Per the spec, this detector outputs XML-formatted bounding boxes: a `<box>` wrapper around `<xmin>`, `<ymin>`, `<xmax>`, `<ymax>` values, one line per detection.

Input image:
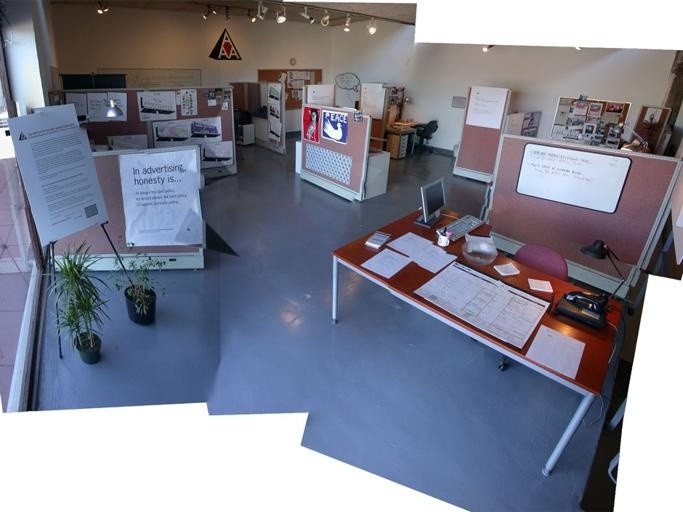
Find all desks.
<box><xmin>385</xmin><ymin>120</ymin><xmax>420</xmax><ymax>158</ymax></box>
<box><xmin>331</xmin><ymin>207</ymin><xmax>623</xmax><ymax>479</ymax></box>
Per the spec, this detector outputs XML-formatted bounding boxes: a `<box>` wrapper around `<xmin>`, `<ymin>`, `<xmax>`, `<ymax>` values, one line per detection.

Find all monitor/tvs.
<box><xmin>412</xmin><ymin>177</ymin><xmax>446</xmax><ymax>228</ymax></box>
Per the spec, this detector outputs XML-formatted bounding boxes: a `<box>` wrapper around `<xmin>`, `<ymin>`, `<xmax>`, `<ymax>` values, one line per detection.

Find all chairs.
<box><xmin>414</xmin><ymin>120</ymin><xmax>437</xmax><ymax>154</ymax></box>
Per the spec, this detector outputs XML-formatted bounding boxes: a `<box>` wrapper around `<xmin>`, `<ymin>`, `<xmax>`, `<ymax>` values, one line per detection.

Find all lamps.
<box><xmin>204</xmin><ymin>0</ymin><xmax>376</xmax><ymax>35</ymax></box>
<box><xmin>77</xmin><ymin>99</ymin><xmax>123</xmax><ymax>121</ymax></box>
<box><xmin>611</xmin><ymin>123</ymin><xmax>648</xmax><ymax>152</ymax></box>
<box><xmin>581</xmin><ymin>241</ymin><xmax>625</xmax><ymax>304</ymax></box>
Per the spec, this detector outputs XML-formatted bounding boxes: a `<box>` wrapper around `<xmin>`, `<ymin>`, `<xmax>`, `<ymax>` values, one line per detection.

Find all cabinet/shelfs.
<box><xmin>238</xmin><ymin>124</ymin><xmax>255</xmax><ymax>145</ymax></box>
<box><xmin>386</xmin><ymin>134</ymin><xmax>408</xmax><ymax>160</ymax></box>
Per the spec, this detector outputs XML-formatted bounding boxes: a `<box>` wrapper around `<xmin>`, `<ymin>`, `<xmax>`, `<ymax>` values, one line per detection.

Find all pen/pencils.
<box><xmin>436</xmin><ymin>226</ymin><xmax>453</xmax><ymax>237</ymax></box>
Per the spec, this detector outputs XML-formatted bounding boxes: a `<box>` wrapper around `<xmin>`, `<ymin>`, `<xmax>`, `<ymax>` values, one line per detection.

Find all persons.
<box><xmin>306</xmin><ymin>110</ymin><xmax>319</xmax><ymax>140</ymax></box>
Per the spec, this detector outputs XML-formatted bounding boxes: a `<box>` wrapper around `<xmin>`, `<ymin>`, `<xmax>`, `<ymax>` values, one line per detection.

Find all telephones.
<box><xmin>555</xmin><ymin>290</ymin><xmax>606</xmax><ymax>330</ymax></box>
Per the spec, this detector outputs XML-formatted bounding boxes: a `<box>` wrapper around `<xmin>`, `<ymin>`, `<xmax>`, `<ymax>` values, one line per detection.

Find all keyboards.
<box><xmin>436</xmin><ymin>214</ymin><xmax>485</xmax><ymax>242</ymax></box>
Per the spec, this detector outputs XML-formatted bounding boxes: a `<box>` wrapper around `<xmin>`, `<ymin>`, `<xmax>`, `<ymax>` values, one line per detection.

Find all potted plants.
<box><xmin>41</xmin><ymin>236</ymin><xmax>114</xmax><ymax>364</ymax></box>
<box><xmin>104</xmin><ymin>236</ymin><xmax>177</xmax><ymax>324</ymax></box>
